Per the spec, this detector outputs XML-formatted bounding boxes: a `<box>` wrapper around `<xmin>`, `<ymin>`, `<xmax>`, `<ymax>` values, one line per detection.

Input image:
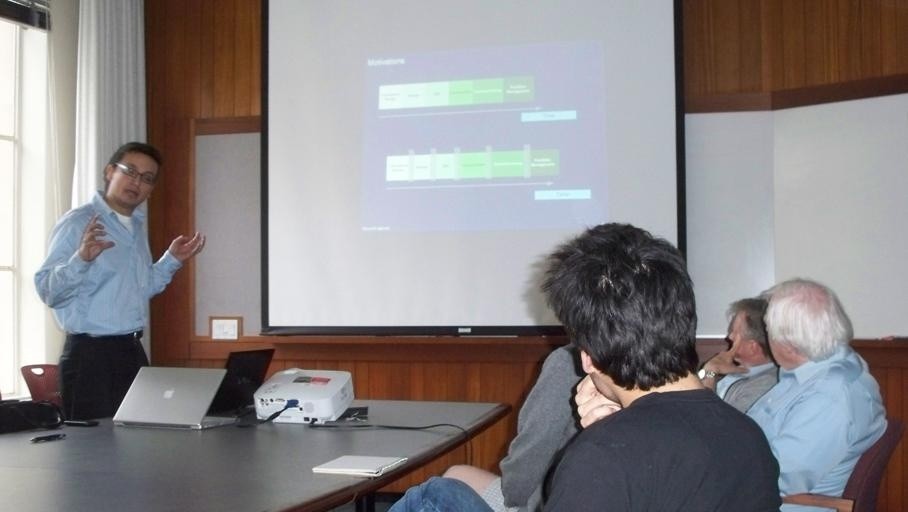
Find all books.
<box><xmin>313</xmin><ymin>455</ymin><xmax>411</xmax><ymax>480</ymax></box>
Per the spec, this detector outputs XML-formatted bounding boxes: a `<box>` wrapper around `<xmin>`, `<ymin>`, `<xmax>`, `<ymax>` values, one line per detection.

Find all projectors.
<box><xmin>253</xmin><ymin>368</ymin><xmax>354</xmax><ymax>425</ymax></box>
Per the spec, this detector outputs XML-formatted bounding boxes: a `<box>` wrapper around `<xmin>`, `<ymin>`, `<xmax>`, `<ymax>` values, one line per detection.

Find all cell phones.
<box><xmin>63</xmin><ymin>419</ymin><xmax>101</xmax><ymax>427</ymax></box>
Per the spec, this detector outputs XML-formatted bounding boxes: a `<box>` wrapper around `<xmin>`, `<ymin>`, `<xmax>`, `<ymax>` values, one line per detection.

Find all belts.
<box><xmin>131</xmin><ymin>330</ymin><xmax>143</xmax><ymax>341</ymax></box>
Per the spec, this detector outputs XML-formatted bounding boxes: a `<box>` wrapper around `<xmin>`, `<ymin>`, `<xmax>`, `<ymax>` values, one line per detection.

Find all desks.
<box><xmin>0</xmin><ymin>395</ymin><xmax>512</xmax><ymax>510</ymax></box>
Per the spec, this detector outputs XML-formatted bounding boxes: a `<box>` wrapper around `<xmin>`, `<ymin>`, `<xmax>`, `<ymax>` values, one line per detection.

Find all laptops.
<box><xmin>210</xmin><ymin>348</ymin><xmax>275</xmax><ymax>413</ymax></box>
<box><xmin>112</xmin><ymin>367</ymin><xmax>240</xmax><ymax>429</ymax></box>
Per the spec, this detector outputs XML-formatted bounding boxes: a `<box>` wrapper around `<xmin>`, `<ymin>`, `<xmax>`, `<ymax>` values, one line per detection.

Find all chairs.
<box><xmin>777</xmin><ymin>420</ymin><xmax>904</xmax><ymax>512</ymax></box>
<box><xmin>21</xmin><ymin>363</ymin><xmax>61</xmax><ymax>406</ymax></box>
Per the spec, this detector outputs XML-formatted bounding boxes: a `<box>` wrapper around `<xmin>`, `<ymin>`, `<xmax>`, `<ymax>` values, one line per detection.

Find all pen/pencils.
<box><xmin>30</xmin><ymin>433</ymin><xmax>66</xmax><ymax>442</ymax></box>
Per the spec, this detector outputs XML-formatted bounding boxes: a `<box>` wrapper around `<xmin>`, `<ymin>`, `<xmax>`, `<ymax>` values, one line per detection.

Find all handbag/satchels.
<box><xmin>0</xmin><ymin>400</ymin><xmax>65</xmax><ymax>434</ymax></box>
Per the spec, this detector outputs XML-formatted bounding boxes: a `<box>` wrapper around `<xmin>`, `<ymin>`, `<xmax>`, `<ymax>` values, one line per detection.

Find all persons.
<box><xmin>388</xmin><ymin>219</ymin><xmax>781</xmax><ymax>512</ymax></box>
<box><xmin>33</xmin><ymin>142</ymin><xmax>207</xmax><ymax>420</ymax></box>
<box><xmin>697</xmin><ymin>279</ymin><xmax>887</xmax><ymax>512</ymax></box>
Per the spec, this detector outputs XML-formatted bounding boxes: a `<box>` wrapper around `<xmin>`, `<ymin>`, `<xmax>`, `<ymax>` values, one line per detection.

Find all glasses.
<box><xmin>115</xmin><ymin>162</ymin><xmax>155</xmax><ymax>185</ymax></box>
